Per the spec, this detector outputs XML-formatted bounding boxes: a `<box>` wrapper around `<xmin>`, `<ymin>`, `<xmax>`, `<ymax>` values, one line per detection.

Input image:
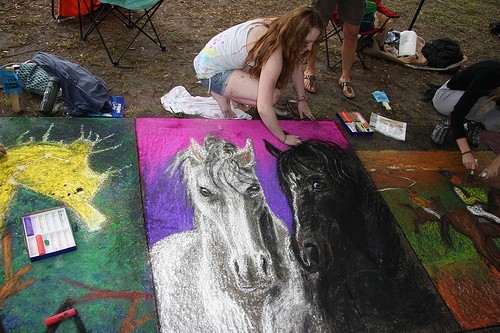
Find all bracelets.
<box><xmin>282</xmin><ymin>135</ymin><xmax>287</xmax><ymax>143</ymax></box>
<box><xmin>297</xmin><ymin>96</ymin><xmax>308</xmax><ymax>102</ymax></box>
<box><xmin>461</xmin><ymin>151</ymin><xmax>472</xmax><ymax>156</ymax></box>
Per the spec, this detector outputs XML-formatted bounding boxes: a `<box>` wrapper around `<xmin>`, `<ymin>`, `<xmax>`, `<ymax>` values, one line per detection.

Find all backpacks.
<box><xmin>420</xmin><ymin>37</ymin><xmax>464</xmax><ymax>68</ymax></box>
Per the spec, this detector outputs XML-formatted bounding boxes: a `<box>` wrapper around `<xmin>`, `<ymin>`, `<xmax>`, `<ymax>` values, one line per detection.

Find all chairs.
<box><xmin>52</xmin><ymin>0</ymin><xmax>166</xmax><ymax>66</ymax></box>
<box><xmin>317</xmin><ymin>0</ymin><xmax>401</xmax><ymax>70</ymax></box>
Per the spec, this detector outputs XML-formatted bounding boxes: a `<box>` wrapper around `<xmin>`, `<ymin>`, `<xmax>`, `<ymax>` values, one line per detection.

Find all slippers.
<box><xmin>303</xmin><ymin>69</ymin><xmax>317</xmax><ymax>93</ymax></box>
<box><xmin>338</xmin><ymin>80</ymin><xmax>355</xmax><ymax>98</ymax></box>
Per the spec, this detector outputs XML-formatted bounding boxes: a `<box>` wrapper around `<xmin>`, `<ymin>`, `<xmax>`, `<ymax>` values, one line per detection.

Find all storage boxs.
<box><xmin>87</xmin><ymin>96</ymin><xmax>124</xmax><ymax>118</ymax></box>
<box><xmin>337</xmin><ymin>112</ymin><xmax>374</xmax><ymax>136</ymax></box>
<box><xmin>20</xmin><ymin>206</ymin><xmax>77</xmax><ymax>262</ymax></box>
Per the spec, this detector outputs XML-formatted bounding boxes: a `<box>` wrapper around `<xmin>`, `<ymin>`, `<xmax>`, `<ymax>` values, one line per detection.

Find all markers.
<box><xmin>45</xmin><ymin>308</ymin><xmax>77</xmax><ymax>327</ymax></box>
<box><xmin>36</xmin><ymin>235</ymin><xmax>45</xmax><ymax>254</ymax></box>
<box><xmin>341</xmin><ymin>111</ymin><xmax>369</xmax><ymax>132</ymax></box>
<box><xmin>470</xmin><ymin>158</ymin><xmax>477</xmax><ymax>175</ymax></box>
<box><xmin>24</xmin><ymin>217</ymin><xmax>34</xmax><ymax>236</ymax></box>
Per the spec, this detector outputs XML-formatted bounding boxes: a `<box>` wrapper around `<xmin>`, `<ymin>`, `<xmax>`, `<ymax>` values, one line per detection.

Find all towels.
<box><xmin>162</xmin><ymin>85</ymin><xmax>251</xmax><ymax>120</ymax></box>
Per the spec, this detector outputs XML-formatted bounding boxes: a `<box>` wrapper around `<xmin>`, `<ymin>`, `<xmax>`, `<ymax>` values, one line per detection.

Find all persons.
<box><xmin>192</xmin><ymin>5</ymin><xmax>329</xmax><ymax>146</ymax></box>
<box><xmin>300</xmin><ymin>0</ymin><xmax>372</xmax><ymax>98</ymax></box>
<box><xmin>433</xmin><ymin>60</ymin><xmax>500</xmax><ymax>182</ymax></box>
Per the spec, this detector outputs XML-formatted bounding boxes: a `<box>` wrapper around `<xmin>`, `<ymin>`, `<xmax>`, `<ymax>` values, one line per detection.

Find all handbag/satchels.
<box><xmin>18</xmin><ymin>62</ymin><xmax>63</xmax><ymax>97</ymax></box>
